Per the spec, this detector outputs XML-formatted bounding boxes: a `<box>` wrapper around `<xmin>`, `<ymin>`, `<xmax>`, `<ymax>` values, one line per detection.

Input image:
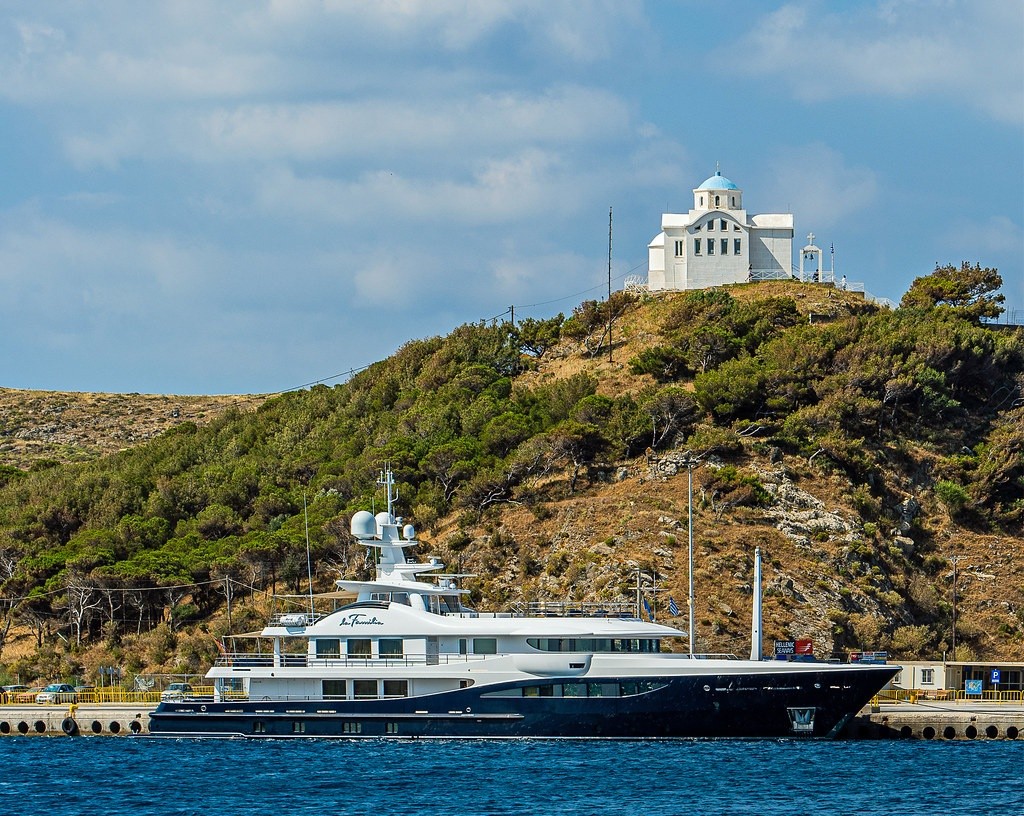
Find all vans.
<box><xmin>2</xmin><ymin>685</ymin><xmax>31</xmax><ymax>701</ymax></box>
<box><xmin>73</xmin><ymin>685</ymin><xmax>96</xmax><ymax>695</ymax></box>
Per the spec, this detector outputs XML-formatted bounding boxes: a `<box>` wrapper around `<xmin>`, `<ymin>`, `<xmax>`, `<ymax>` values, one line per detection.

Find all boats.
<box><xmin>142</xmin><ymin>457</ymin><xmax>903</xmax><ymax>740</ymax></box>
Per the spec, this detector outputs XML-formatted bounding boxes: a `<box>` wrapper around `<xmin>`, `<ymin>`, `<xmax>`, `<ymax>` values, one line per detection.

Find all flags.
<box><xmin>669</xmin><ymin>596</ymin><xmax>678</xmax><ymax>617</ymax></box>
<box><xmin>643</xmin><ymin>598</ymin><xmax>654</xmax><ymax>621</ymax></box>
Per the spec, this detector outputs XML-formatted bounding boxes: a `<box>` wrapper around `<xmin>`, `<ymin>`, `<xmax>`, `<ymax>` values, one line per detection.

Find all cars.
<box><xmin>16</xmin><ymin>686</ymin><xmax>44</xmax><ymax>702</ymax></box>
<box><xmin>160</xmin><ymin>683</ymin><xmax>194</xmax><ymax>702</ymax></box>
<box><xmin>36</xmin><ymin>684</ymin><xmax>79</xmax><ymax>705</ymax></box>
